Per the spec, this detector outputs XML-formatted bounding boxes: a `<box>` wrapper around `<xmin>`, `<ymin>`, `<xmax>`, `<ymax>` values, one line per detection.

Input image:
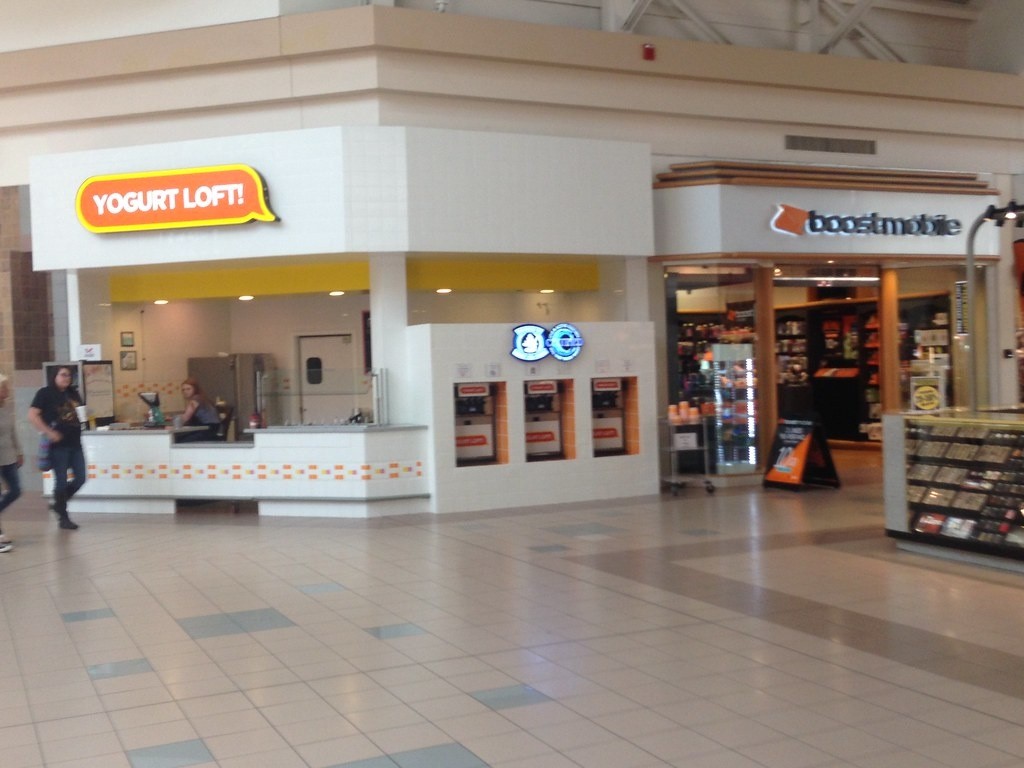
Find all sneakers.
<box><xmin>0</xmin><ymin>542</ymin><xmax>13</xmax><ymax>552</ymax></box>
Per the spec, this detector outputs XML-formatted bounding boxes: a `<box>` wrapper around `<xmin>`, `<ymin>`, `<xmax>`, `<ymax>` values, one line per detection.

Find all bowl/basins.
<box><xmin>668</xmin><ymin>401</ymin><xmax>701</xmax><ymax>424</ymax></box>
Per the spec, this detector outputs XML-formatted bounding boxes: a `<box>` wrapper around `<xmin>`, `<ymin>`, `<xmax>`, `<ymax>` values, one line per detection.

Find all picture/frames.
<box><xmin>120</xmin><ymin>331</ymin><xmax>134</xmax><ymax>347</ymax></box>
<box><xmin>120</xmin><ymin>351</ymin><xmax>137</xmax><ymax>370</ymax></box>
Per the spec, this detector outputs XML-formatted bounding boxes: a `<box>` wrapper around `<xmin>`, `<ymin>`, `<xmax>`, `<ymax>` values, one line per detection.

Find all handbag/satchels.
<box><xmin>38</xmin><ymin>432</ymin><xmax>52</xmax><ymax>471</ymax></box>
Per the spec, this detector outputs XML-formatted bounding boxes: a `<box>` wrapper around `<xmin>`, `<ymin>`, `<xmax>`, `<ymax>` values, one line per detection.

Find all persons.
<box><xmin>164</xmin><ymin>378</ymin><xmax>219</xmax><ymax>443</ymax></box>
<box><xmin>28</xmin><ymin>364</ymin><xmax>85</xmax><ymax>529</ymax></box>
<box><xmin>0</xmin><ymin>375</ymin><xmax>24</xmax><ymax>552</ymax></box>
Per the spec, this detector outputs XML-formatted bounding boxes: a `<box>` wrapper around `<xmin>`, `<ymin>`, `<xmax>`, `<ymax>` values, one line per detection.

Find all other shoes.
<box><xmin>54</xmin><ymin>503</ymin><xmax>69</xmax><ymax>519</ymax></box>
<box><xmin>59</xmin><ymin>521</ymin><xmax>77</xmax><ymax>529</ymax></box>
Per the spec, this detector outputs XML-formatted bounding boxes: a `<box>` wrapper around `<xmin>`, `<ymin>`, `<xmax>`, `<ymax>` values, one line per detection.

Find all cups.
<box><xmin>247</xmin><ymin>415</ymin><xmax>260</xmax><ymax>428</ymax></box>
<box><xmin>75</xmin><ymin>405</ymin><xmax>88</xmax><ymax>423</ymax></box>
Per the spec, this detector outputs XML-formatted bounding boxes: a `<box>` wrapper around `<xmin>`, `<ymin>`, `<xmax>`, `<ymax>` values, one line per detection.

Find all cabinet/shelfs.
<box><xmin>659</xmin><ymin>417</ymin><xmax>715</xmax><ymax>495</ymax></box>
